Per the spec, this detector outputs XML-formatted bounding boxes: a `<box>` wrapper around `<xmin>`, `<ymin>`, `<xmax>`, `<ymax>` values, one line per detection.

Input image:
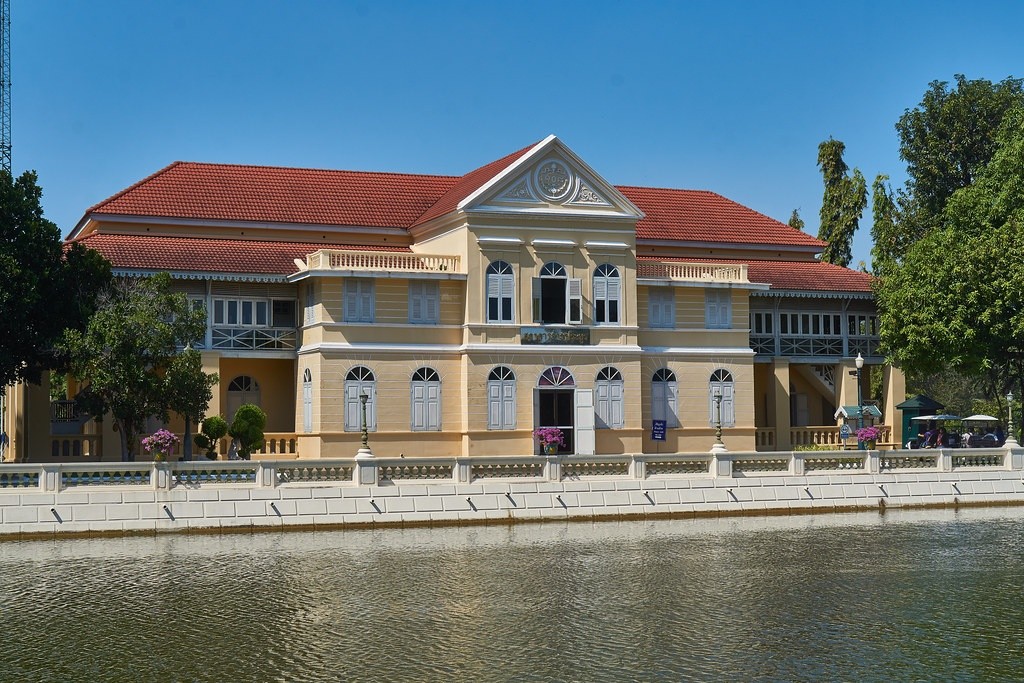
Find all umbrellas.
<box><xmin>912</xmin><ymin>414</ymin><xmax>961</xmax><ymax>420</ymax></box>
<box><xmin>961</xmin><ymin>414</ymin><xmax>998</xmax><ymax>421</ymax></box>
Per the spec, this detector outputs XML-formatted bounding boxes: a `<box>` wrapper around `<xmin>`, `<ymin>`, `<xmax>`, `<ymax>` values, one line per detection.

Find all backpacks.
<box><xmin>965</xmin><ymin>432</ymin><xmax>982</xmax><ymax>448</ymax></box>
<box><xmin>982</xmin><ymin>434</ymin><xmax>996</xmax><ymax>448</ymax></box>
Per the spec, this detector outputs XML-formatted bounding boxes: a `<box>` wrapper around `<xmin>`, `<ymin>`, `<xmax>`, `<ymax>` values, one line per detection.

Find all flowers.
<box><xmin>532</xmin><ymin>428</ymin><xmax>566</xmax><ymax>448</ymax></box>
<box><xmin>141</xmin><ymin>428</ymin><xmax>180</xmax><ymax>457</ymax></box>
<box><xmin>855</xmin><ymin>427</ymin><xmax>882</xmax><ymax>442</ymax></box>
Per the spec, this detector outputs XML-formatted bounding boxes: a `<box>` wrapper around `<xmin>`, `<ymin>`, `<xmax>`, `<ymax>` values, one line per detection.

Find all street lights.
<box><xmin>855</xmin><ymin>351</ymin><xmax>865</xmax><ymax>450</ymax></box>
<box><xmin>183</xmin><ymin>342</ymin><xmax>194</xmax><ymax>459</ymax></box>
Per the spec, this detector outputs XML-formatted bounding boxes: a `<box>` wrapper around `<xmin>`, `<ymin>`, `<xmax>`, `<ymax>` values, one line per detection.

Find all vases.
<box><xmin>154</xmin><ymin>452</ymin><xmax>167</xmax><ymax>461</ymax></box>
<box><xmin>861</xmin><ymin>440</ymin><xmax>876</xmax><ymax>450</ymax></box>
<box><xmin>542</xmin><ymin>442</ymin><xmax>558</xmax><ymax>455</ymax></box>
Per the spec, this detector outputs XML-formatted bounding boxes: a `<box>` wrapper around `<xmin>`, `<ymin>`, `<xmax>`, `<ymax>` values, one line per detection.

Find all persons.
<box><xmin>923</xmin><ymin>422</ymin><xmax>958</xmax><ymax>447</ymax></box>
<box><xmin>964</xmin><ymin>426</ymin><xmax>1004</xmax><ymax>447</ymax></box>
